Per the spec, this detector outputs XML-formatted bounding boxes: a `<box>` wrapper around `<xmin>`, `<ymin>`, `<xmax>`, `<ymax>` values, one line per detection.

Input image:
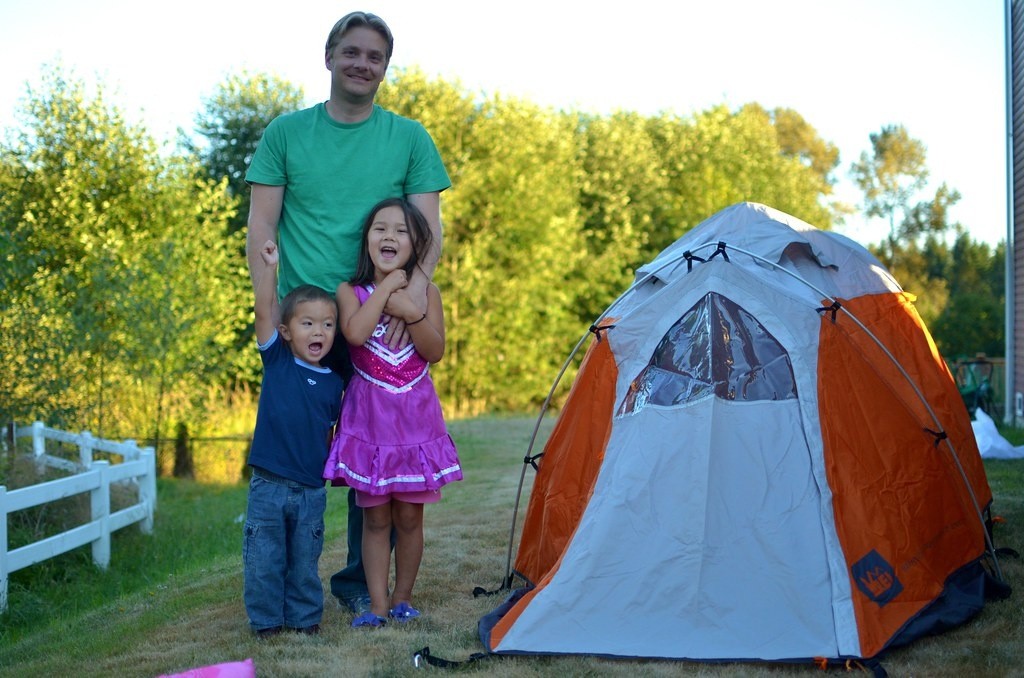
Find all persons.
<box><xmin>241</xmin><ymin>239</ymin><xmax>346</xmax><ymax>641</ymax></box>
<box><xmin>241</xmin><ymin>12</ymin><xmax>451</xmax><ymax>614</ymax></box>
<box><xmin>317</xmin><ymin>195</ymin><xmax>462</xmax><ymax>635</ymax></box>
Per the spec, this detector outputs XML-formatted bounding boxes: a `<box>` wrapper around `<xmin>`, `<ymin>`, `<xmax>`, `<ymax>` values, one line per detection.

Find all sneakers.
<box><xmin>293</xmin><ymin>623</ymin><xmax>323</xmax><ymax>636</ymax></box>
<box><xmin>256</xmin><ymin>626</ymin><xmax>281</xmax><ymax>640</ymax></box>
<box><xmin>345</xmin><ymin>595</ymin><xmax>371</xmax><ymax>617</ymax></box>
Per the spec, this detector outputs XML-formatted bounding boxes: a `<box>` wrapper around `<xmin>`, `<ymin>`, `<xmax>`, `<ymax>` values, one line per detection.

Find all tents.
<box><xmin>474</xmin><ymin>198</ymin><xmax>1012</xmax><ymax>668</ymax></box>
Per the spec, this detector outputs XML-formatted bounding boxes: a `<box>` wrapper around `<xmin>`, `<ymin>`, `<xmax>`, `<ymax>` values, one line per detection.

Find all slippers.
<box><xmin>351</xmin><ymin>612</ymin><xmax>387</xmax><ymax>628</ymax></box>
<box><xmin>388</xmin><ymin>603</ymin><xmax>420</xmax><ymax>624</ymax></box>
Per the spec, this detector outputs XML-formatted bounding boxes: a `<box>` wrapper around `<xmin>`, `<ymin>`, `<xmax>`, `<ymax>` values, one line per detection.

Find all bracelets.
<box><xmin>406</xmin><ymin>313</ymin><xmax>426</xmax><ymax>326</ymax></box>
